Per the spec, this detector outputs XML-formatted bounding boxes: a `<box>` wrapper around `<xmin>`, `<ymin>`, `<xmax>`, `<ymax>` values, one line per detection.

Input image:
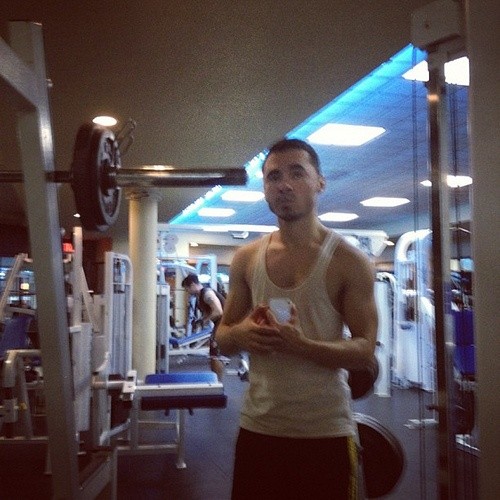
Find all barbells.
<box><xmin>1</xmin><ymin>118</ymin><xmax>247</xmax><ymax>232</ymax></box>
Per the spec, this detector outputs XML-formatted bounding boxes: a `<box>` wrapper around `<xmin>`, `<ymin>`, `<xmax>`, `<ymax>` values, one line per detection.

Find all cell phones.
<box><xmin>268</xmin><ymin>296</ymin><xmax>292</xmax><ymax>323</ymax></box>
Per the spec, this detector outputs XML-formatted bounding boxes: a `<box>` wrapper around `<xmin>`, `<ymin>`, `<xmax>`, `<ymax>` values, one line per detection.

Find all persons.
<box><xmin>0</xmin><ymin>301</ymin><xmax>32</xmax><ymax>406</ymax></box>
<box><xmin>182</xmin><ymin>272</ymin><xmax>226</xmax><ymax>383</ymax></box>
<box><xmin>215</xmin><ymin>137</ymin><xmax>379</xmax><ymax>500</ymax></box>
